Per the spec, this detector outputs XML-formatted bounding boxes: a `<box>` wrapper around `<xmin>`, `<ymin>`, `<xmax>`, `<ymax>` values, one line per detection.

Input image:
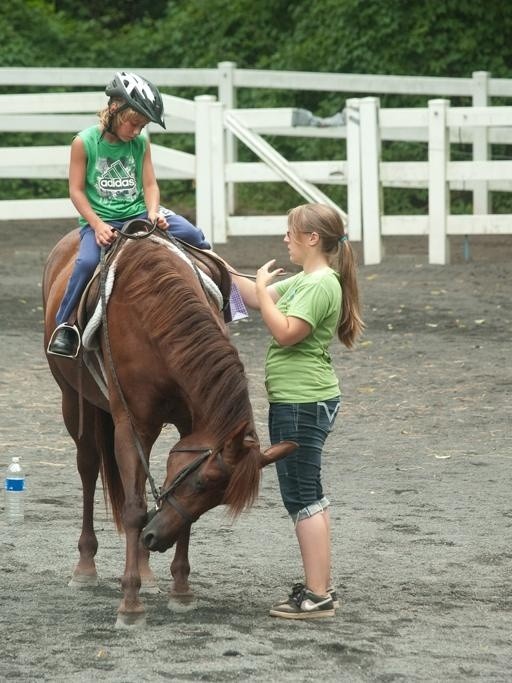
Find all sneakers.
<box><xmin>270</xmin><ymin>589</ymin><xmax>338</xmax><ymax>619</ymax></box>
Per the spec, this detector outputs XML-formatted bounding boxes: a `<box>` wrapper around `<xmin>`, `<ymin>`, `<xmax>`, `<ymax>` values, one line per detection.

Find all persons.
<box><xmin>49</xmin><ymin>70</ymin><xmax>214</xmax><ymax>357</ymax></box>
<box><xmin>194</xmin><ymin>203</ymin><xmax>368</xmax><ymax>618</ymax></box>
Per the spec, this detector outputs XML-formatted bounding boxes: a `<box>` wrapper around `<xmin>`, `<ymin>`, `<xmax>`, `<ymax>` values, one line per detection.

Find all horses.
<box><xmin>41</xmin><ymin>224</ymin><xmax>300</xmax><ymax>632</ymax></box>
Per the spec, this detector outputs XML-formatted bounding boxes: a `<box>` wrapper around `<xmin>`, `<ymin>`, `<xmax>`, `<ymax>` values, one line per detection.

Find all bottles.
<box><xmin>463</xmin><ymin>233</ymin><xmax>471</xmax><ymax>263</ymax></box>
<box><xmin>5</xmin><ymin>456</ymin><xmax>25</xmax><ymax>523</ymax></box>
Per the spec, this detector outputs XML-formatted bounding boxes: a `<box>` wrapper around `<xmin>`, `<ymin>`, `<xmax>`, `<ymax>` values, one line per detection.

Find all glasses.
<box><xmin>287</xmin><ymin>231</ymin><xmax>313</xmax><ymax>238</ymax></box>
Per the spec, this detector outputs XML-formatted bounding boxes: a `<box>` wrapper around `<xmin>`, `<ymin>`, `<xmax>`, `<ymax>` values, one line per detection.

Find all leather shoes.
<box><xmin>51</xmin><ymin>329</ymin><xmax>75</xmax><ymax>355</ymax></box>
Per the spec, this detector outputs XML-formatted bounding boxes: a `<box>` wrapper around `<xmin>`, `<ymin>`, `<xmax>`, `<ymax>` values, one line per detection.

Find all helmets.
<box><xmin>105</xmin><ymin>72</ymin><xmax>166</xmax><ymax>130</ymax></box>
